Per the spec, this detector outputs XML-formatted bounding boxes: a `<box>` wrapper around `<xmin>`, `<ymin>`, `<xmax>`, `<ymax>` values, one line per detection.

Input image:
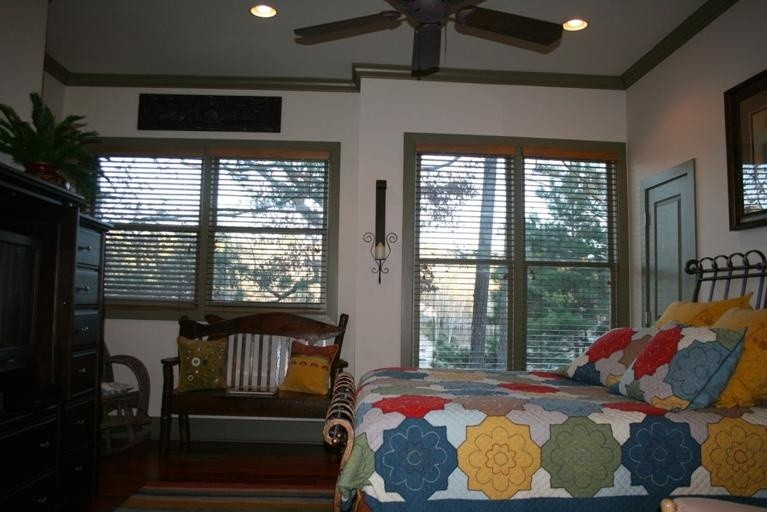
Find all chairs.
<box><xmin>98</xmin><ymin>344</ymin><xmax>152</xmax><ymax>455</ymax></box>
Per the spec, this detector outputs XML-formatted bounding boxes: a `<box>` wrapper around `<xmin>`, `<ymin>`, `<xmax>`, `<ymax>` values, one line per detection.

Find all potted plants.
<box><xmin>0</xmin><ymin>91</ymin><xmax>111</xmax><ymax>188</ymax></box>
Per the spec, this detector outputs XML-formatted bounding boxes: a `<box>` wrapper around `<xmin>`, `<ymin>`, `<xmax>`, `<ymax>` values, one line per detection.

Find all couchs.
<box><xmin>161</xmin><ymin>313</ymin><xmax>349</xmax><ymax>454</ymax></box>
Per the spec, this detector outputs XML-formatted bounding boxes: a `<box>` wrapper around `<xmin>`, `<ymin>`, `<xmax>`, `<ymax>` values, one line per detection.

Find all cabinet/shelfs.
<box><xmin>1</xmin><ymin>403</ymin><xmax>60</xmax><ymax>510</ymax></box>
<box><xmin>60</xmin><ymin>213</ymin><xmax>116</xmax><ymax>511</ymax></box>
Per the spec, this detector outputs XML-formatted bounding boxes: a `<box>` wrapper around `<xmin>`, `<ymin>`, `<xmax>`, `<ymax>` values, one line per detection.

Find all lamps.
<box><xmin>562</xmin><ymin>17</ymin><xmax>590</xmax><ymax>33</ymax></box>
<box><xmin>247</xmin><ymin>3</ymin><xmax>279</xmax><ymax>19</ymax></box>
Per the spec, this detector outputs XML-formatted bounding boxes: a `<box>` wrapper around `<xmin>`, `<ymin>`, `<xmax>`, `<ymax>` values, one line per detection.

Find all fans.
<box><xmin>294</xmin><ymin>0</ymin><xmax>562</xmax><ymax>77</ymax></box>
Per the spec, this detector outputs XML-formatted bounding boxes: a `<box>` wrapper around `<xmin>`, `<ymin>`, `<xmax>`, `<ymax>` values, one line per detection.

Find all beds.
<box><xmin>323</xmin><ymin>251</ymin><xmax>767</xmax><ymax>512</ymax></box>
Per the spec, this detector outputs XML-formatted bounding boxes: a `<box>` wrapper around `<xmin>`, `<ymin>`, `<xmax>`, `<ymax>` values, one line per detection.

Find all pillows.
<box><xmin>557</xmin><ymin>327</ymin><xmax>659</xmax><ymax>388</ymax></box>
<box><xmin>655</xmin><ymin>292</ymin><xmax>754</xmax><ymax>327</ymax></box>
<box><xmin>176</xmin><ymin>336</ymin><xmax>229</xmax><ymax>392</ymax></box>
<box><xmin>607</xmin><ymin>321</ymin><xmax>748</xmax><ymax>413</ymax></box>
<box><xmin>711</xmin><ymin>307</ymin><xmax>767</xmax><ymax>408</ymax></box>
<box><xmin>280</xmin><ymin>340</ymin><xmax>338</xmax><ymax>396</ymax></box>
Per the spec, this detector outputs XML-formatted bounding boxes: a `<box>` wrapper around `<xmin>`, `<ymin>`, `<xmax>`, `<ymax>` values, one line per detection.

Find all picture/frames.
<box><xmin>724</xmin><ymin>69</ymin><xmax>767</xmax><ymax>231</ymax></box>
